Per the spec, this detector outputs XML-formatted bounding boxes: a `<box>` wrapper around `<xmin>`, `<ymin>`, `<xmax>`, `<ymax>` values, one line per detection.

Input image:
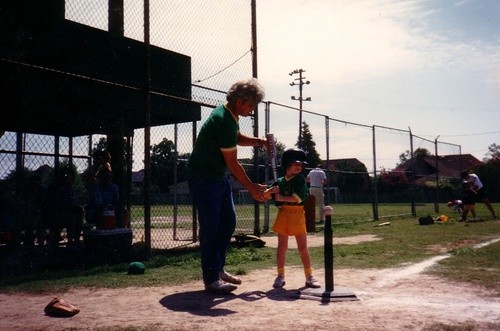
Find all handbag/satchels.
<box><xmin>419</xmin><ymin>215</ymin><xmax>435</xmax><ymax>225</ymax></box>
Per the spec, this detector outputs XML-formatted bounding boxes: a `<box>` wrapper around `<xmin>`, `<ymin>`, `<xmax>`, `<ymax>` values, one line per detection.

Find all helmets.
<box><xmin>281</xmin><ymin>149</ymin><xmax>308</xmax><ymax>176</ymax></box>
<box><xmin>127</xmin><ymin>261</ymin><xmax>146</xmax><ymax>276</ymax></box>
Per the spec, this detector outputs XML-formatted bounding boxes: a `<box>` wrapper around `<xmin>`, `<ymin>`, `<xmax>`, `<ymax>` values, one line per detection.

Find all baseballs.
<box><xmin>324</xmin><ymin>206</ymin><xmax>333</xmax><ymax>215</ymax></box>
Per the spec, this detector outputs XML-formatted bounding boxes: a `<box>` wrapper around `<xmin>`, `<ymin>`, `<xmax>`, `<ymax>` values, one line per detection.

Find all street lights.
<box><xmin>289</xmin><ymin>69</ymin><xmax>311</xmax><ymax>148</ymax></box>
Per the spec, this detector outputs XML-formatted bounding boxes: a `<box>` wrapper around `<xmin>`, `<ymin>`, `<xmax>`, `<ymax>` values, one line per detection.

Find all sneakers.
<box><xmin>273</xmin><ymin>277</ymin><xmax>285</xmax><ymax>287</ymax></box>
<box><xmin>306</xmin><ymin>274</ymin><xmax>320</xmax><ymax>288</ymax></box>
<box><xmin>219</xmin><ymin>271</ymin><xmax>242</xmax><ymax>284</ymax></box>
<box><xmin>205</xmin><ymin>279</ymin><xmax>237</xmax><ymax>294</ymax></box>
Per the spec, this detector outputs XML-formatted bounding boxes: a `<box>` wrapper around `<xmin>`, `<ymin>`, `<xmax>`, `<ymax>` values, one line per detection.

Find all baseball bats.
<box><xmin>266</xmin><ymin>133</ymin><xmax>282</xmax><ymax>210</ymax></box>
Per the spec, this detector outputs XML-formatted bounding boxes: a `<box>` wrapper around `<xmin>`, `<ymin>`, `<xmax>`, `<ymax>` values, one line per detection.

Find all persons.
<box><xmin>305</xmin><ymin>163</ymin><xmax>328</xmax><ymax>222</ymax></box>
<box><xmin>262</xmin><ymin>147</ymin><xmax>321</xmax><ymax>288</ymax></box>
<box><xmin>32</xmin><ymin>164</ymin><xmax>53</xmax><ymax>182</ymax></box>
<box><xmin>447</xmin><ymin>199</ymin><xmax>463</xmax><ymax>215</ymax></box>
<box><xmin>82</xmin><ymin>149</ymin><xmax>114</xmax><ymax>186</ymax></box>
<box><xmin>460</xmin><ymin>181</ymin><xmax>478</xmax><ymax>222</ymax></box>
<box><xmin>185</xmin><ymin>76</ymin><xmax>277</xmax><ymax>295</ymax></box>
<box><xmin>85</xmin><ymin>163</ymin><xmax>124</xmax><ymax>225</ymax></box>
<box><xmin>458</xmin><ymin>170</ymin><xmax>497</xmax><ymax>223</ymax></box>
